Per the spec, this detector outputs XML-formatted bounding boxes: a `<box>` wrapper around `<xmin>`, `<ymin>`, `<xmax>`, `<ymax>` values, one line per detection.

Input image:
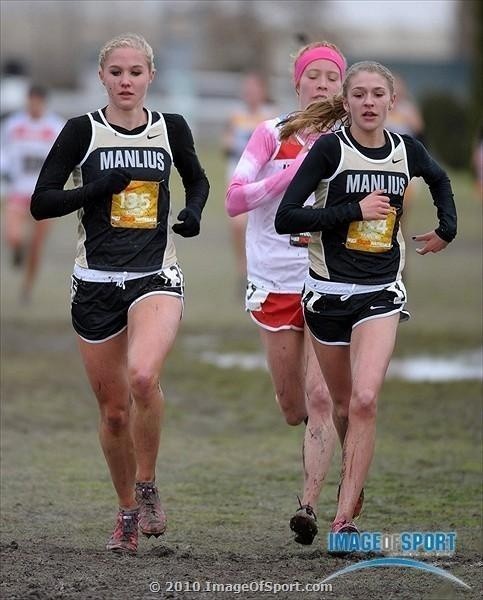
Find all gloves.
<box><xmin>172</xmin><ymin>209</ymin><xmax>201</xmax><ymax>237</ymax></box>
<box><xmin>93</xmin><ymin>168</ymin><xmax>131</xmax><ymax>194</ymax></box>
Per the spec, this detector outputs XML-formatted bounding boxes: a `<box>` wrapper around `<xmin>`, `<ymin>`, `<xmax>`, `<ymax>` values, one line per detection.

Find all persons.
<box><xmin>216</xmin><ymin>72</ymin><xmax>283</xmax><ymax>291</ymax></box>
<box><xmin>221</xmin><ymin>40</ymin><xmax>356</xmax><ymax>551</ymax></box>
<box><xmin>1</xmin><ymin>82</ymin><xmax>70</xmax><ymax>307</ymax></box>
<box><xmin>269</xmin><ymin>57</ymin><xmax>460</xmax><ymax>560</ymax></box>
<box><xmin>383</xmin><ymin>76</ymin><xmax>425</xmax><ymax>230</ymax></box>
<box><xmin>28</xmin><ymin>31</ymin><xmax>211</xmax><ymax>558</ymax></box>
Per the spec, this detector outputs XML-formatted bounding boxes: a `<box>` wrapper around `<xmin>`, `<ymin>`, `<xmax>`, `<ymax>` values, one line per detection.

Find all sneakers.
<box><xmin>106</xmin><ymin>510</ymin><xmax>138</xmax><ymax>556</ymax></box>
<box><xmin>290</xmin><ymin>509</ymin><xmax>317</xmax><ymax>545</ymax></box>
<box><xmin>135</xmin><ymin>481</ymin><xmax>166</xmax><ymax>538</ymax></box>
<box><xmin>330</xmin><ymin>522</ymin><xmax>359</xmax><ymax>555</ymax></box>
<box><xmin>338</xmin><ymin>485</ymin><xmax>364</xmax><ymax>519</ymax></box>
